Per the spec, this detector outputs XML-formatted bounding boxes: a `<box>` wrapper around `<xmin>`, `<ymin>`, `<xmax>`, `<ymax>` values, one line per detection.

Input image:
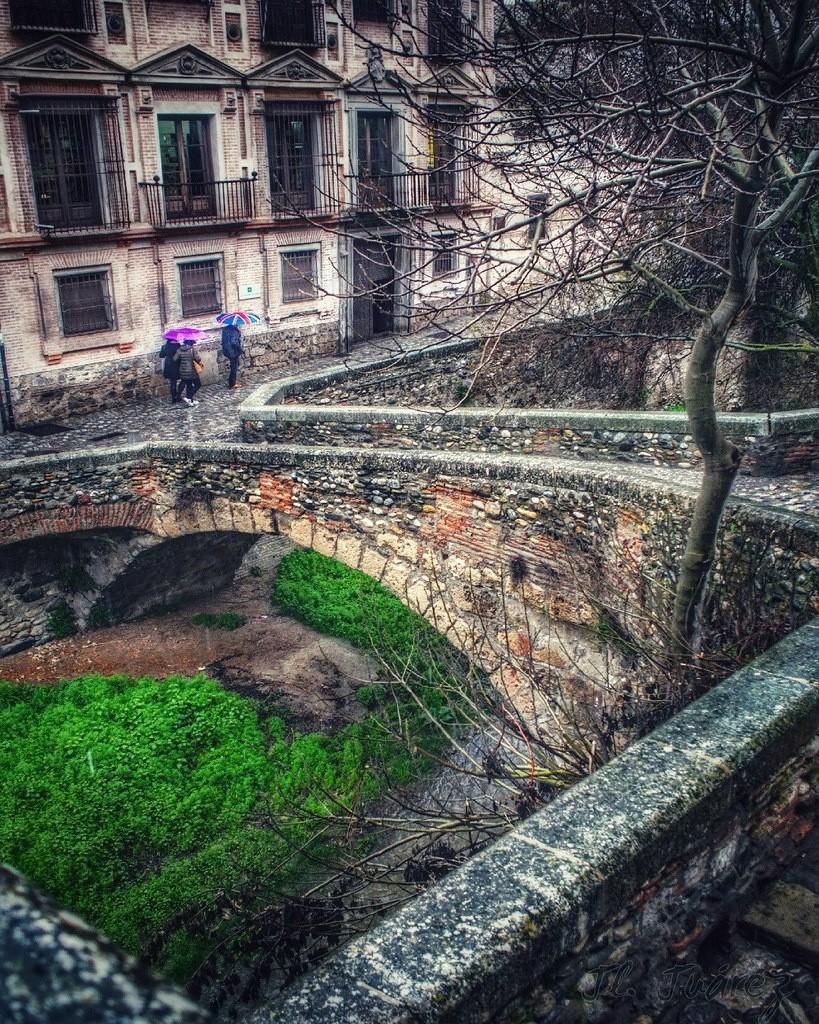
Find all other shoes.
<box><xmin>193</xmin><ymin>400</ymin><xmax>199</xmax><ymax>405</ymax></box>
<box><xmin>172</xmin><ymin>400</ymin><xmax>176</xmax><ymax>405</ymax></box>
<box><xmin>231</xmin><ymin>384</ymin><xmax>241</xmax><ymax>388</ymax></box>
<box><xmin>183</xmin><ymin>398</ymin><xmax>194</xmax><ymax>406</ymax></box>
<box><xmin>177</xmin><ymin>397</ymin><xmax>182</xmax><ymax>402</ymax></box>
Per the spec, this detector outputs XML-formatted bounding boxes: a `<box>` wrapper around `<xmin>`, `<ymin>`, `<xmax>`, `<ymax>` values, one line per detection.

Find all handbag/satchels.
<box><xmin>191</xmin><ymin>348</ymin><xmax>203</xmax><ymax>373</ymax></box>
<box><xmin>162</xmin><ymin>345</ymin><xmax>166</xmax><ymax>373</ymax></box>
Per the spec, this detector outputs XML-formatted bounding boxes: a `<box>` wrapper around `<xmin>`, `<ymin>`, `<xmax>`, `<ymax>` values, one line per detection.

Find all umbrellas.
<box><xmin>163</xmin><ymin>327</ymin><xmax>206</xmax><ymax>342</ymax></box>
<box><xmin>216</xmin><ymin>312</ymin><xmax>260</xmax><ymax>325</ymax></box>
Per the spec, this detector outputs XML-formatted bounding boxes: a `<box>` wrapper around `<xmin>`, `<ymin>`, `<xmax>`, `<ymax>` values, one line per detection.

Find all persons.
<box><xmin>173</xmin><ymin>340</ymin><xmax>201</xmax><ymax>406</ymax></box>
<box><xmin>159</xmin><ymin>339</ymin><xmax>186</xmax><ymax>402</ymax></box>
<box><xmin>221</xmin><ymin>325</ymin><xmax>243</xmax><ymax>387</ymax></box>
